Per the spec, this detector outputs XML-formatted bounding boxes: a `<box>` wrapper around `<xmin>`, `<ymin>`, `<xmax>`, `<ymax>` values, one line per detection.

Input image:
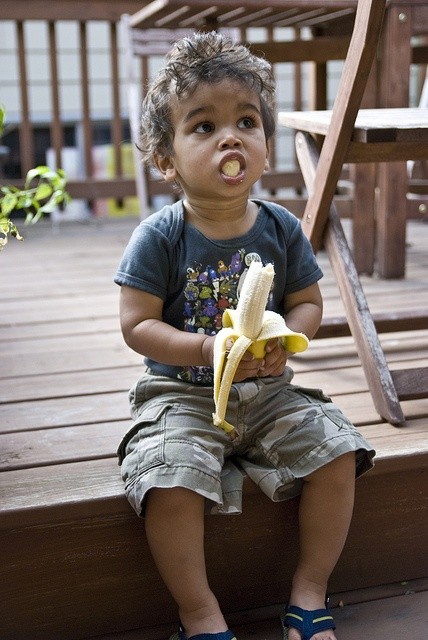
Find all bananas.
<box><xmin>210</xmin><ymin>258</ymin><xmax>310</xmax><ymax>442</ymax></box>
<box><xmin>220</xmin><ymin>158</ymin><xmax>242</xmax><ymax>178</ymax></box>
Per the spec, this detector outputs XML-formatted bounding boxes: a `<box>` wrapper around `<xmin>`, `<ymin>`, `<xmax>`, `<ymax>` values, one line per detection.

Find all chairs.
<box><xmin>277</xmin><ymin>0</ymin><xmax>428</xmax><ymax>426</ymax></box>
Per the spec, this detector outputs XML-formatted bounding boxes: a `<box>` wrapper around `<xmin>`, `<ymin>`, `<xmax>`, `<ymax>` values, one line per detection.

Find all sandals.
<box><xmin>281</xmin><ymin>604</ymin><xmax>335</xmax><ymax>640</ymax></box>
<box><xmin>178</xmin><ymin>626</ymin><xmax>237</xmax><ymax>639</ymax></box>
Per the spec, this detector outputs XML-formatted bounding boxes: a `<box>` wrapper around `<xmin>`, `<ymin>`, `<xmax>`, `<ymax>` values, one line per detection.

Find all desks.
<box><xmin>118</xmin><ymin>0</ymin><xmax>428</xmax><ymax>278</ymax></box>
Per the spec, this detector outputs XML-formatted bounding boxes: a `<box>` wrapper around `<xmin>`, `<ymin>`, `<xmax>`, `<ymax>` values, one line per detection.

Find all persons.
<box><xmin>113</xmin><ymin>31</ymin><xmax>378</xmax><ymax>639</ymax></box>
